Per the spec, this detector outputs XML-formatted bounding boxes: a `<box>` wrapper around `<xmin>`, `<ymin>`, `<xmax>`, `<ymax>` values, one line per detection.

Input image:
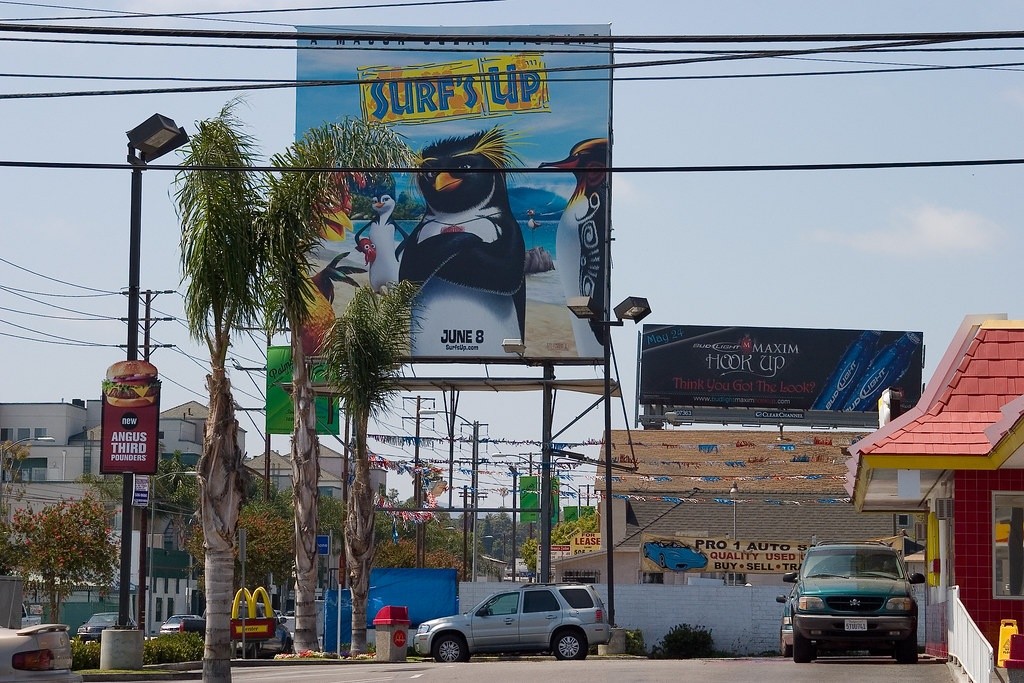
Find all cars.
<box><xmin>202</xmin><ymin>599</ymin><xmax>293</xmax><ymax>658</ymax></box>
<box><xmin>0</xmin><ymin>623</ymin><xmax>84</xmax><ymax>682</ymax></box>
<box><xmin>76</xmin><ymin>612</ymin><xmax>139</xmax><ymax>641</ymax></box>
<box><xmin>160</xmin><ymin>614</ymin><xmax>207</xmax><ymax>637</ymax></box>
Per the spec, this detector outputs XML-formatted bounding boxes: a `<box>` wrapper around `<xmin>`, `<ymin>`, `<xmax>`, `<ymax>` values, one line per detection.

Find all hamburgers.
<box><xmin>101</xmin><ymin>360</ymin><xmax>162</xmax><ymax>407</ymax></box>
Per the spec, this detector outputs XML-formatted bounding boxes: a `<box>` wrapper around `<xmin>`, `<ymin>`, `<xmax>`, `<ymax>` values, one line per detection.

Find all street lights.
<box><xmin>566</xmin><ymin>295</ymin><xmax>653</xmax><ymax>657</ymax></box>
<box><xmin>0</xmin><ymin>437</ymin><xmax>55</xmax><ymax>513</ymax></box>
<box><xmin>147</xmin><ymin>471</ymin><xmax>199</xmax><ymax>639</ymax></box>
<box><xmin>491</xmin><ymin>452</ymin><xmax>541</xmax><ymax>584</ymax></box>
<box><xmin>417</xmin><ymin>409</ymin><xmax>478</xmax><ymax>581</ymax></box>
<box><xmin>559</xmin><ymin>482</ymin><xmax>581</xmax><ymax>519</ymax></box>
<box><xmin>97</xmin><ymin>112</ymin><xmax>191</xmax><ymax>669</ymax></box>
<box><xmin>729</xmin><ymin>480</ymin><xmax>739</xmax><ymax>588</ymax></box>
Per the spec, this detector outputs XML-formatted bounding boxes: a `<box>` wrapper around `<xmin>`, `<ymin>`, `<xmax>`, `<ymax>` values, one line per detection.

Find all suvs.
<box><xmin>413</xmin><ymin>581</ymin><xmax>612</xmax><ymax>663</ymax></box>
<box><xmin>776</xmin><ymin>538</ymin><xmax>926</xmax><ymax>664</ymax></box>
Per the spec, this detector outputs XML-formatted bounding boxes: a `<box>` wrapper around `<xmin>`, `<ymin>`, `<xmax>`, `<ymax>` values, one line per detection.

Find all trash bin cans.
<box><xmin>372</xmin><ymin>605</ymin><xmax>411</xmax><ymax>662</ymax></box>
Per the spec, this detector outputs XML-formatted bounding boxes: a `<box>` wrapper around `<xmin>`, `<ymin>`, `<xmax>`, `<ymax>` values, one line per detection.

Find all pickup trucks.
<box><xmin>21</xmin><ymin>603</ymin><xmax>42</xmax><ymax>628</ymax></box>
